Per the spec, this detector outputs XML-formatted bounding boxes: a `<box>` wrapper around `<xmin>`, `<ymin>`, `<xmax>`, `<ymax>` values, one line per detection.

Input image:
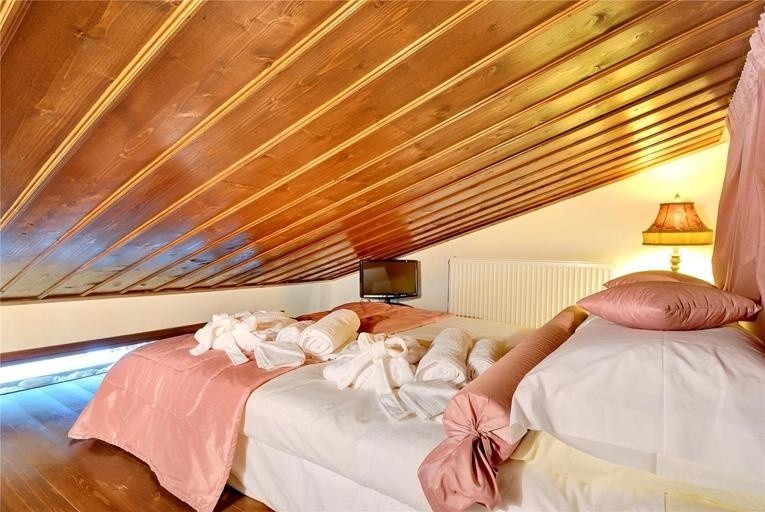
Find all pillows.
<box><xmin>575</xmin><ymin>281</ymin><xmax>762</xmax><ymax>331</ymax></box>
<box><xmin>602</xmin><ymin>269</ymin><xmax>720</xmax><ymax>289</ymax></box>
<box><xmin>508</xmin><ymin>312</ymin><xmax>765</xmax><ymax>508</ymax></box>
<box><xmin>417</xmin><ymin>305</ymin><xmax>589</xmax><ymax>509</ymax></box>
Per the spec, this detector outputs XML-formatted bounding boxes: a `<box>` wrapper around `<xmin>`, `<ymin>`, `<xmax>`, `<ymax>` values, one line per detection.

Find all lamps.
<box><xmin>640</xmin><ymin>203</ymin><xmax>715</xmax><ymax>276</ymax></box>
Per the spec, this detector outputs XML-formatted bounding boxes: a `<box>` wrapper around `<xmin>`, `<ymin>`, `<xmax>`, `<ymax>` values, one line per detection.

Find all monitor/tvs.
<box><xmin>359</xmin><ymin>259</ymin><xmax>418</xmax><ymax>308</ymax></box>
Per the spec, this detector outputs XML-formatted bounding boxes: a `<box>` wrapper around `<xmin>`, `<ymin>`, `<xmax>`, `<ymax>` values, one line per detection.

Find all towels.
<box><xmin>277</xmin><ymin>309</ymin><xmax>498</xmax><ymax>407</ymax></box>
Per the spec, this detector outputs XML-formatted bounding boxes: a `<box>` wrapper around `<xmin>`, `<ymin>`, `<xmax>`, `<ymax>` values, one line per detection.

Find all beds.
<box><xmin>69</xmin><ymin>301</ymin><xmax>765</xmax><ymax>509</ymax></box>
